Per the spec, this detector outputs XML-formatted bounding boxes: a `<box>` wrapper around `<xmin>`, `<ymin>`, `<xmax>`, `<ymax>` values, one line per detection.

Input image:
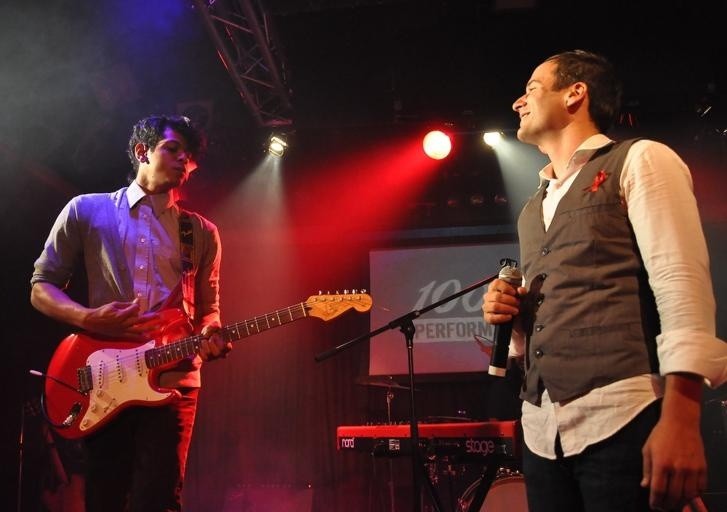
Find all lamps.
<box><xmin>259</xmin><ymin>130</ymin><xmax>291</xmax><ymax>162</ymax></box>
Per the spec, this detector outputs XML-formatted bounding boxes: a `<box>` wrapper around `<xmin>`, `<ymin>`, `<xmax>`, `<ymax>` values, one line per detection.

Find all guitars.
<box><xmin>45</xmin><ymin>288</ymin><xmax>373</xmax><ymax>440</ymax></box>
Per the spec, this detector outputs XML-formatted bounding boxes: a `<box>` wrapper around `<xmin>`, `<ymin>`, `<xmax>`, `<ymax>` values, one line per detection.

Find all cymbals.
<box><xmin>355</xmin><ymin>378</ymin><xmax>419</xmax><ymax>393</ymax></box>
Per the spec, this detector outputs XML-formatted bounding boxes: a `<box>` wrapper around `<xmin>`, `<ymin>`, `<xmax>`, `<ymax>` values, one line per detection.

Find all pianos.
<box><xmin>336</xmin><ymin>415</ymin><xmax>523</xmax><ymax>466</ymax></box>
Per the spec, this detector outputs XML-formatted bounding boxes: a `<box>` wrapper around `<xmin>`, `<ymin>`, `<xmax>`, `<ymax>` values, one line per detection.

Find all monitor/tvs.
<box><xmin>358</xmin><ymin>233</ymin><xmax>528</xmax><ymax>386</ymax></box>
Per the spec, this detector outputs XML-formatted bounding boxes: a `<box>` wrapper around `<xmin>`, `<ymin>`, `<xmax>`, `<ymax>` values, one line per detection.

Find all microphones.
<box><xmin>29</xmin><ymin>369</ymin><xmax>80</xmax><ymax>393</ymax></box>
<box><xmin>488</xmin><ymin>264</ymin><xmax>523</xmax><ymax>381</ymax></box>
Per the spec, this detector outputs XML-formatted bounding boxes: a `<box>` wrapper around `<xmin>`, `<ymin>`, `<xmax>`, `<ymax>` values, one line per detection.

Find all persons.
<box><xmin>26</xmin><ymin>115</ymin><xmax>234</xmax><ymax>510</ymax></box>
<box><xmin>479</xmin><ymin>48</ymin><xmax>726</xmax><ymax>510</ymax></box>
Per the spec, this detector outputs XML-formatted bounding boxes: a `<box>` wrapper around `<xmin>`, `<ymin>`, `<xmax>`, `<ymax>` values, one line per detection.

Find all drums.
<box><xmin>455</xmin><ymin>464</ymin><xmax>530</xmax><ymax>512</ymax></box>
